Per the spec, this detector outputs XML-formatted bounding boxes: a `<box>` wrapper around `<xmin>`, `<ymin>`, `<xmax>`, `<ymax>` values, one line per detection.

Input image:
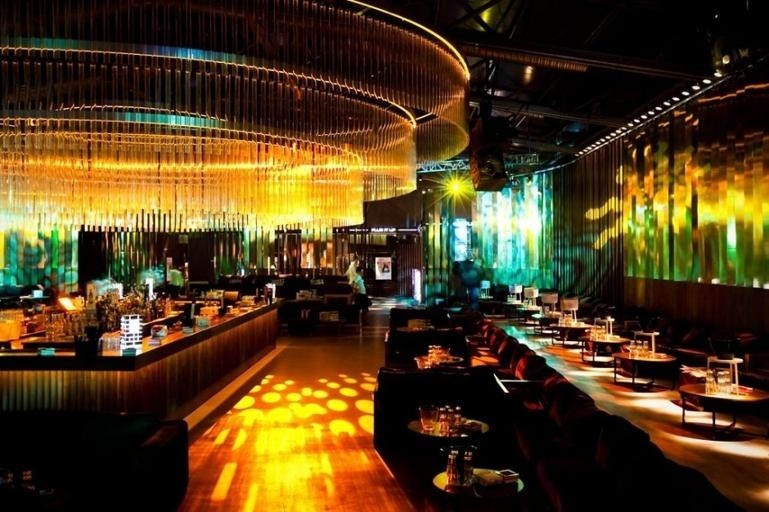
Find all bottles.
<box><xmin>144</xmin><ymin>295</ymin><xmax>173</xmax><ymax>323</ymax></box>
<box><xmin>45</xmin><ymin>311</ymin><xmax>88</xmax><ymax>338</ymax></box>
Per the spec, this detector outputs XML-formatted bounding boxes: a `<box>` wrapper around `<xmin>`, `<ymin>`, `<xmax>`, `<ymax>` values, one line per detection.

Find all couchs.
<box><xmin>1</xmin><ymin>405</ymin><xmax>190</xmax><ymax>509</ymax></box>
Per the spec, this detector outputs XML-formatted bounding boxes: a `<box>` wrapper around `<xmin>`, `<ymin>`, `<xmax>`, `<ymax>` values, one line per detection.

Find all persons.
<box><xmin>347</xmin><ymin>254</ymin><xmax>373</xmax><ymax>309</ymax></box>
<box><xmin>355</xmin><ymin>267</ymin><xmax>371</xmax><ymax>328</ymax></box>
